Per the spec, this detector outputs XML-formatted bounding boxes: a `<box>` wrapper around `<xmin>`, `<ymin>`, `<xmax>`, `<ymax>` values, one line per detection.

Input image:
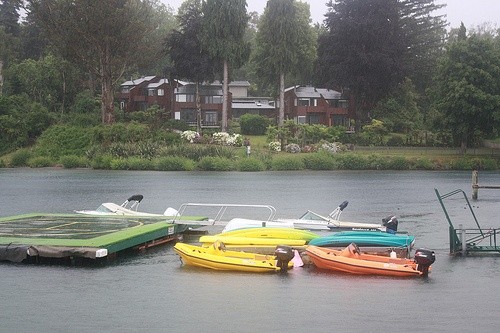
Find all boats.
<box><xmin>73</xmin><ymin>195</ymin><xmax>183</xmax><ymax>218</ymax></box>
<box><xmin>310</xmin><ymin>215</ymin><xmax>417</xmax><ymax>251</ymax></box>
<box><xmin>173</xmin><ymin>241</ymin><xmax>298</xmax><ymax>273</ymax></box>
<box><xmin>197</xmin><ymin>226</ymin><xmax>319</xmax><ymax>250</ymax></box>
<box><xmin>273</xmin><ymin>200</ymin><xmax>407</xmax><ymax>234</ymax></box>
<box><xmin>306</xmin><ymin>243</ymin><xmax>436</xmax><ymax>277</ymax></box>
